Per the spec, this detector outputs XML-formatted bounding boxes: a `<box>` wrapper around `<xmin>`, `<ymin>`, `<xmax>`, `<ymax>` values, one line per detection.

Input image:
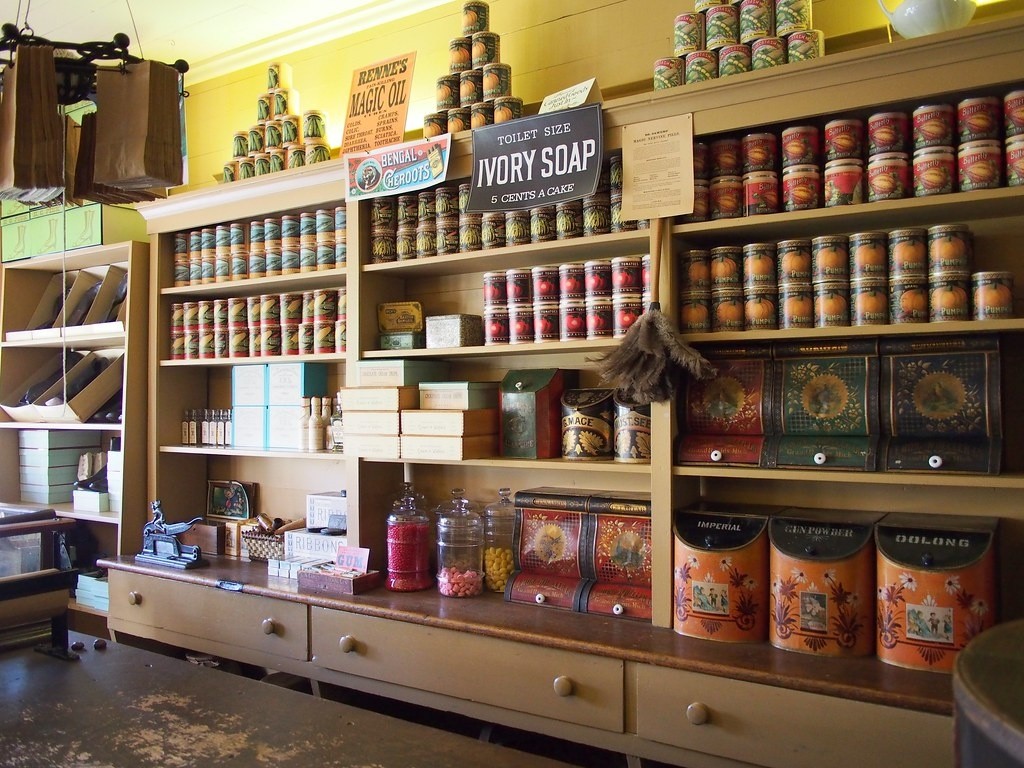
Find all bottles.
<box><xmin>181</xmin><ymin>408</ymin><xmax>231</xmax><ymax>447</ymax></box>
<box><xmin>297</xmin><ymin>392</ymin><xmax>343</xmax><ymax>452</ymax></box>
<box><xmin>384</xmin><ymin>481</ymin><xmax>516</xmax><ymax>598</ymax></box>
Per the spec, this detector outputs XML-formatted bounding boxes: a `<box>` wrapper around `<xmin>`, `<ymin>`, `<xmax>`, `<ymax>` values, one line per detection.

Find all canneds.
<box><xmin>423</xmin><ymin>0</ymin><xmax>523</xmax><ymax>140</ymax></box>
<box><xmin>169</xmin><ymin>206</ymin><xmax>348</xmax><ymax>359</ymax></box>
<box><xmin>678</xmin><ymin>224</ymin><xmax>1016</xmax><ymax>335</ymax></box>
<box><xmin>219</xmin><ymin>61</ymin><xmax>331</xmax><ymax>182</ymax></box>
<box><xmin>369</xmin><ymin>157</ymin><xmax>650</xmax><ymax>265</ymax></box>
<box><xmin>483</xmin><ymin>252</ymin><xmax>651</xmax><ymax>346</ymax></box>
<box><xmin>677</xmin><ymin>88</ymin><xmax>1024</xmax><ymax>224</ymax></box>
<box><xmin>654</xmin><ymin>0</ymin><xmax>826</xmax><ymax>90</ymax></box>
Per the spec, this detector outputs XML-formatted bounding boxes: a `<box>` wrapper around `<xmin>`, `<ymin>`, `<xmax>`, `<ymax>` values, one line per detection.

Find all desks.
<box><xmin>951</xmin><ymin>621</ymin><xmax>1024</xmax><ymax>768</ymax></box>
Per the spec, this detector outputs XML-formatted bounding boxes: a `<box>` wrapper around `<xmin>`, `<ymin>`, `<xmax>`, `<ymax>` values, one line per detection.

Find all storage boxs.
<box><xmin>5</xmin><ymin>261</ymin><xmax>128</xmax><ymax>342</ymax></box>
<box><xmin>0</xmin><ymin>348</ymin><xmax>126</xmax><ymax>423</ymax></box>
<box><xmin>73</xmin><ymin>451</ymin><xmax>122</xmax><ymax>512</ymax></box>
<box><xmin>62</xmin><ymin>566</ymin><xmax>109</xmax><ymax>612</ymax></box>
<box><xmin>231</xmin><ymin>362</ymin><xmax>328</xmax><ymax>449</ymax></box>
<box><xmin>504</xmin><ymin>486</ymin><xmax>652</xmax><ymax>620</ymax></box>
<box><xmin>377</xmin><ymin>300</ymin><xmax>484</xmax><ymax>350</ymax></box>
<box><xmin>18</xmin><ymin>429</ymin><xmax>103</xmax><ymax>505</ymax></box>
<box><xmin>672</xmin><ymin>339</ymin><xmax>1012</xmax><ymax>476</ymax></box>
<box><xmin>672</xmin><ymin>500</ymin><xmax>1002</xmax><ymax>673</ymax></box>
<box><xmin>1</xmin><ymin>196</ymin><xmax>151</xmax><ymax>263</ymax></box>
<box><xmin>171</xmin><ymin>517</ymin><xmax>259</xmax><ymax>557</ymax></box>
<box><xmin>498</xmin><ymin>368</ymin><xmax>651</xmax><ymax>463</ymax></box>
<box><xmin>339</xmin><ymin>360</ymin><xmax>501</xmax><ymax>461</ymax></box>
<box><xmin>268</xmin><ymin>492</ymin><xmax>380</xmax><ymax>595</ymax></box>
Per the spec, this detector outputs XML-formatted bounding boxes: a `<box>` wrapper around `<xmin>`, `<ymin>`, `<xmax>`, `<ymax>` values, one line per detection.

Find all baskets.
<box><xmin>241</xmin><ymin>528</ymin><xmax>285</xmax><ymax>561</ymax></box>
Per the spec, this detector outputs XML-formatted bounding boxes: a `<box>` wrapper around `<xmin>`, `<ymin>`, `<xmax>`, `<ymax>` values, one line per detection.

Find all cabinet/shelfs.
<box><xmin>0</xmin><ymin>240</ymin><xmax>150</xmax><ymax>642</ymax></box>
<box><xmin>95</xmin><ymin>11</ymin><xmax>1023</xmax><ymax>768</ymax></box>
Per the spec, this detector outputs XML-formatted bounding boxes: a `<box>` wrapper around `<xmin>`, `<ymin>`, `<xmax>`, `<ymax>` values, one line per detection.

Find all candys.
<box><xmin>438</xmin><ymin>546</ymin><xmax>515</xmax><ymax>597</ymax></box>
